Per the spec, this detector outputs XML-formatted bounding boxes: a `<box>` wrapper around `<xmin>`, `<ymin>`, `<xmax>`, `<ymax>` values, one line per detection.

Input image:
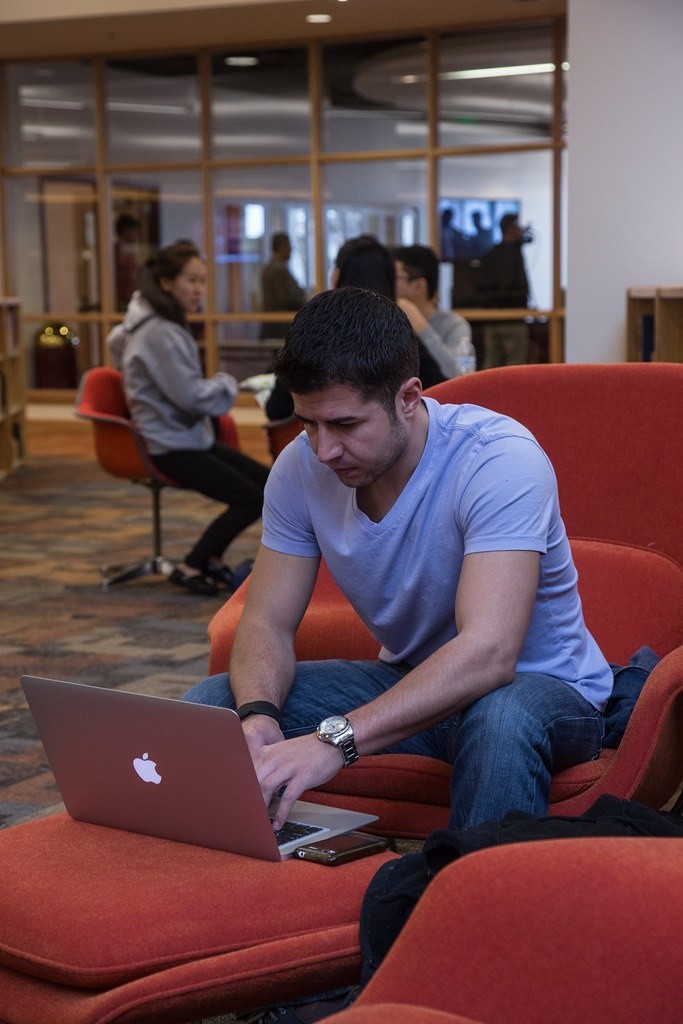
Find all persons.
<box><xmin>104</xmin><ymin>215</ymin><xmax>271</xmax><ymax>599</ymax></box>
<box><xmin>175</xmin><ymin>287</ymin><xmax>614</xmax><ymax>874</ymax></box>
<box><xmin>332</xmin><ymin>206</ymin><xmax>530</xmax><ymax>389</ymax></box>
<box><xmin>231</xmin><ymin>793</ymin><xmax>683</xmax><ymax>1024</ymax></box>
<box><xmin>260</xmin><ymin>233</ymin><xmax>306</xmax><ymax>343</ymax></box>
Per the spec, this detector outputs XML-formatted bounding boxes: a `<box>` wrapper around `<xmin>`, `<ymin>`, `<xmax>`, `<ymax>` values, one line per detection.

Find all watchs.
<box><xmin>316</xmin><ymin>714</ymin><xmax>360</xmax><ymax>768</ymax></box>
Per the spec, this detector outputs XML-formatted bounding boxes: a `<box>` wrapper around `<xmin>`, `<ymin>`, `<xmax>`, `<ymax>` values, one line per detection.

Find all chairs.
<box><xmin>206</xmin><ymin>363</ymin><xmax>683</xmax><ymax>838</ymax></box>
<box><xmin>72</xmin><ymin>367</ymin><xmax>242</xmax><ymax>592</ymax></box>
<box><xmin>326</xmin><ymin>840</ymin><xmax>683</xmax><ymax>1024</ymax></box>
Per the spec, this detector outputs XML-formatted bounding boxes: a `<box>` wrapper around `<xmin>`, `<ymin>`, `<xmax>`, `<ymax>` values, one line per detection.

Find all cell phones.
<box><xmin>292</xmin><ymin>831</ymin><xmax>388</xmax><ymax>866</ymax></box>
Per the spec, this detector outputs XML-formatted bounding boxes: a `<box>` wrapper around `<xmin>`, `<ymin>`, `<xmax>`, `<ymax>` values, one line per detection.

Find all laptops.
<box><xmin>20</xmin><ymin>673</ymin><xmax>380</xmax><ymax>861</ymax></box>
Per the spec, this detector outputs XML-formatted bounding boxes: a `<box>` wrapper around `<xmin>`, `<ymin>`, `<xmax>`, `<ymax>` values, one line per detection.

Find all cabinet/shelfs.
<box><xmin>0</xmin><ymin>187</ymin><xmax>31</xmax><ymax>482</ymax></box>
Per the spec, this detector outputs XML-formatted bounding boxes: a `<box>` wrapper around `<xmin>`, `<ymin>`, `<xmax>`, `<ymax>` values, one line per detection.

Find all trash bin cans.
<box><xmin>35</xmin><ymin>323</ymin><xmax>79</xmax><ymax>389</ymax></box>
<box><xmin>480</xmin><ymin>320</ymin><xmax>548</xmax><ymax>369</ymax></box>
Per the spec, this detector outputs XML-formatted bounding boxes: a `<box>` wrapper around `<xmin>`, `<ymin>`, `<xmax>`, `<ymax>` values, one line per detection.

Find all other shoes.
<box><xmin>206</xmin><ymin>559</ymin><xmax>245</xmax><ymax>587</ymax></box>
<box><xmin>169</xmin><ymin>567</ymin><xmax>222</xmax><ymax>598</ymax></box>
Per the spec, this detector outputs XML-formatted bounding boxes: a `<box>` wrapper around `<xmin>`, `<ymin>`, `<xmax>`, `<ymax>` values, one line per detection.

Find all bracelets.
<box><xmin>236</xmin><ymin>700</ymin><xmax>283</xmax><ymax>730</ymax></box>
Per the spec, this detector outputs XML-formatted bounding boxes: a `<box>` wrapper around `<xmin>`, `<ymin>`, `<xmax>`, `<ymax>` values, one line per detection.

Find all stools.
<box><xmin>0</xmin><ymin>801</ymin><xmax>408</xmax><ymax>1024</ymax></box>
